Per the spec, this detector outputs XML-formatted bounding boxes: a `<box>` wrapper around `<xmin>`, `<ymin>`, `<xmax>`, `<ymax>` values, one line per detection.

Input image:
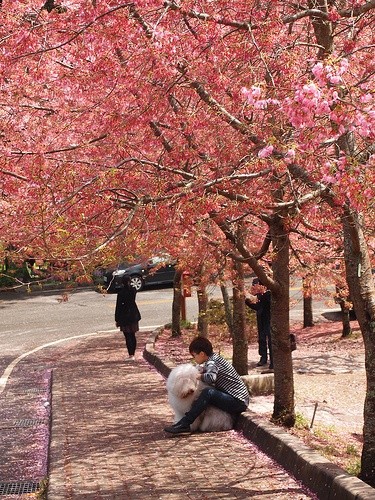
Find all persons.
<box><xmin>244</xmin><ymin>290</ymin><xmax>274</xmax><ymax>374</ymax></box>
<box><xmin>251</xmin><ymin>261</ymin><xmax>273</xmax><ymax>367</ymax></box>
<box><xmin>163</xmin><ymin>337</ymin><xmax>251</xmax><ymax>435</ymax></box>
<box><xmin>115</xmin><ymin>274</ymin><xmax>142</xmax><ymax>362</ymax></box>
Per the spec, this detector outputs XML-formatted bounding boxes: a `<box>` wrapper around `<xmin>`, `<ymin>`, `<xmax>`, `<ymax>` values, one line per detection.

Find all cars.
<box><xmin>103</xmin><ymin>251</ymin><xmax>179</xmax><ymax>293</ymax></box>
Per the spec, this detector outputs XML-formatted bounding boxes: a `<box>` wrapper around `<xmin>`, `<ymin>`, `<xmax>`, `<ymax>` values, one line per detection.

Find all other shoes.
<box><xmin>269</xmin><ymin>359</ymin><xmax>273</xmax><ymax>369</ymax></box>
<box><xmin>258</xmin><ymin>357</ymin><xmax>267</xmax><ymax>365</ymax></box>
<box><xmin>125</xmin><ymin>355</ymin><xmax>135</xmax><ymax>360</ymax></box>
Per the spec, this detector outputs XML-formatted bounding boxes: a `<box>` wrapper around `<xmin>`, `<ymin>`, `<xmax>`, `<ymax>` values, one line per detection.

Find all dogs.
<box><xmin>166</xmin><ymin>362</ymin><xmax>233</xmax><ymax>431</ymax></box>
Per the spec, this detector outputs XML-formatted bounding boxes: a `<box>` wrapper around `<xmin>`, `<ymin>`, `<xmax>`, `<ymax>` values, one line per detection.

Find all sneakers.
<box><xmin>163</xmin><ymin>420</ymin><xmax>192</xmax><ymax>435</ymax></box>
